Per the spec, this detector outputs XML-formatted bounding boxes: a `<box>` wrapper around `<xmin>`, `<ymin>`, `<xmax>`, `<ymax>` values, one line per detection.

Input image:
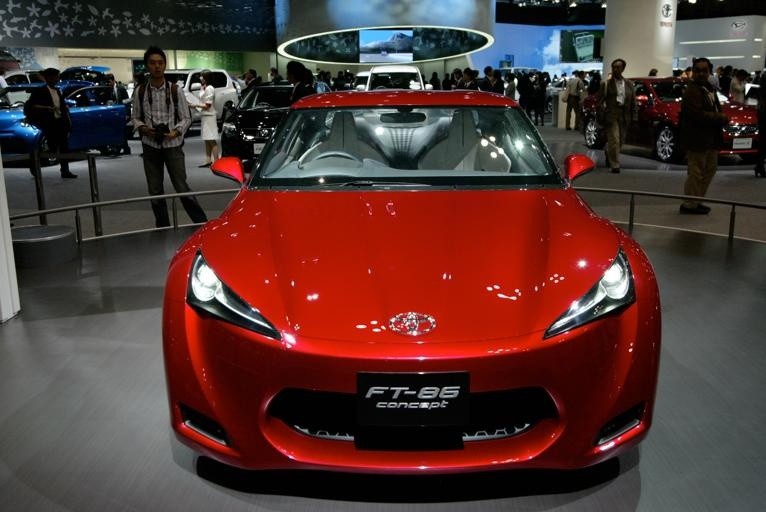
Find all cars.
<box><xmin>221</xmin><ymin>81</ymin><xmax>333</xmax><ymax>160</ymax></box>
<box><xmin>161</xmin><ymin>89</ymin><xmax>663</xmax><ymax>476</ymax></box>
<box><xmin>496</xmin><ymin>67</ymin><xmax>540</xmax><ymax>101</ymax></box>
<box><xmin>0</xmin><ymin>66</ymin><xmax>127</xmax><ymax>167</ymax></box>
<box><xmin>343</xmin><ymin>71</ymin><xmax>370</xmax><ymax>89</ymax></box>
<box><xmin>0</xmin><ymin>69</ymin><xmax>44</xmax><ymax>108</ymax></box>
<box><xmin>546</xmin><ymin>76</ymin><xmax>591</xmax><ymax>113</ymax></box>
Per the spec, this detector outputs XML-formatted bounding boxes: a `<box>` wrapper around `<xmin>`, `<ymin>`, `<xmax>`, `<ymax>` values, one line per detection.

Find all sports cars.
<box><xmin>580</xmin><ymin>76</ymin><xmax>760</xmax><ymax>163</ymax></box>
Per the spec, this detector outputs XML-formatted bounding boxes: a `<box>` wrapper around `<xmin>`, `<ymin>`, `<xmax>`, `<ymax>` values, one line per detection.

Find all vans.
<box><xmin>356</xmin><ymin>65</ymin><xmax>434</xmax><ymax>91</ymax></box>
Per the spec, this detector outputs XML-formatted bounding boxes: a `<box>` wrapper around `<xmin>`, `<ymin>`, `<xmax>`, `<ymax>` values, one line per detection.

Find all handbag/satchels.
<box><xmin>596</xmin><ymin>103</ymin><xmax>618</xmax><ymax>127</ymax></box>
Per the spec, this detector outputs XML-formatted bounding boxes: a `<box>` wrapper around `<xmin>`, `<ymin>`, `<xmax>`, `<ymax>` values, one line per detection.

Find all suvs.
<box><xmin>571</xmin><ymin>32</ymin><xmax>595</xmax><ymax>62</ymax></box>
<box><xmin>162</xmin><ymin>68</ymin><xmax>242</xmax><ymax>129</ymax></box>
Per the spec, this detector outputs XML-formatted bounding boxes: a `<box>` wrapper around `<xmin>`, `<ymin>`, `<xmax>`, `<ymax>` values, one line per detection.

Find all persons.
<box><xmin>37</xmin><ymin>69</ymin><xmax>45</xmax><ymax>83</ymax></box>
<box><xmin>679</xmin><ymin>57</ymin><xmax>729</xmax><ymax>214</ymax></box>
<box><xmin>188</xmin><ymin>70</ymin><xmax>220</xmax><ymax>167</ymax></box>
<box><xmin>754</xmin><ymin>57</ymin><xmax>766</xmax><ymax>176</ymax></box>
<box><xmin>423</xmin><ymin>66</ymin><xmax>516</xmax><ymax>100</ymax></box>
<box><xmin>517</xmin><ymin>70</ymin><xmax>601</xmax><ymax>131</ymax></box>
<box><xmin>133</xmin><ymin>46</ymin><xmax>208</xmax><ymax>227</ymax></box>
<box><xmin>235</xmin><ymin>61</ymin><xmax>355</xmax><ymax>107</ymax></box>
<box><xmin>649</xmin><ymin>69</ymin><xmax>658</xmax><ymax>77</ymax></box>
<box><xmin>133</xmin><ymin>69</ymin><xmax>145</xmax><ymax>157</ymax></box>
<box><xmin>595</xmin><ymin>59</ymin><xmax>638</xmax><ymax>173</ymax></box>
<box><xmin>104</xmin><ymin>73</ymin><xmax>132</xmax><ymax>156</ymax></box>
<box><xmin>23</xmin><ymin>68</ymin><xmax>79</xmax><ymax>179</ymax></box>
<box><xmin>675</xmin><ymin>64</ymin><xmax>760</xmax><ymax>103</ymax></box>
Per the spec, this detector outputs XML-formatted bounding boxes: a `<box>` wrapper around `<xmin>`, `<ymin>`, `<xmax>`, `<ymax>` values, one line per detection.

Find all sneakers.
<box><xmin>612</xmin><ymin>168</ymin><xmax>621</xmax><ymax>173</ymax></box>
<box><xmin>680</xmin><ymin>203</ymin><xmax>710</xmax><ymax>215</ymax></box>
<box><xmin>65</xmin><ymin>173</ymin><xmax>78</xmax><ymax>179</ymax></box>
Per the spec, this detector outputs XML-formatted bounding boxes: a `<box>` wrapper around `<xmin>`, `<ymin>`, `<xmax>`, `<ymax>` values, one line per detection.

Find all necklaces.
<box><xmin>0</xmin><ymin>63</ymin><xmax>12</xmax><ymax>106</ymax></box>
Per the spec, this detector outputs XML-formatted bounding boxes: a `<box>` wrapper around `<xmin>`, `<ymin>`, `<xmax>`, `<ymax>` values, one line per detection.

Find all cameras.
<box><xmin>154</xmin><ymin>123</ymin><xmax>170</xmax><ymax>145</ymax></box>
